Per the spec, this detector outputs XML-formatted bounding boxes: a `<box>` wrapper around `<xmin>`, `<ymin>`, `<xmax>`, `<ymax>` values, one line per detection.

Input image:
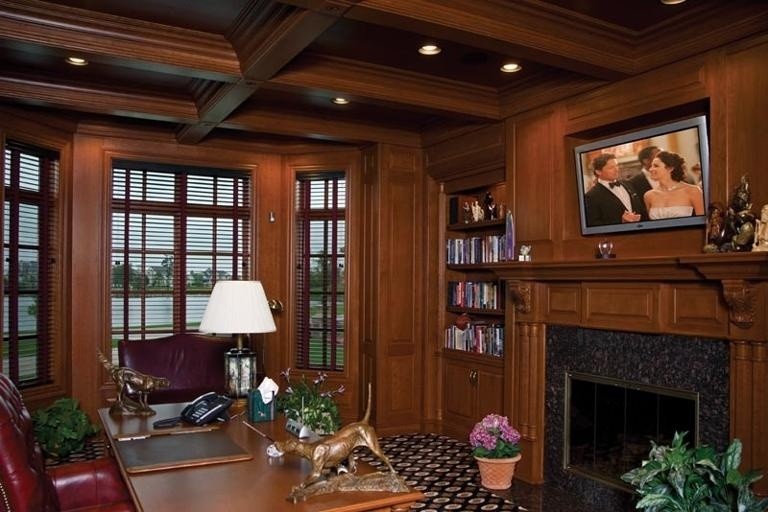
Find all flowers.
<box><xmin>275</xmin><ymin>367</ymin><xmax>345</xmax><ymax>432</ymax></box>
<box><xmin>468</xmin><ymin>413</ymin><xmax>523</xmax><ymax>459</ymax></box>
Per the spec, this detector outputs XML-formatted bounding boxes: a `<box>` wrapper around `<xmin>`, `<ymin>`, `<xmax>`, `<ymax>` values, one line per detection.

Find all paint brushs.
<box><xmin>243</xmin><ymin>421</ymin><xmax>276</xmax><ymax>442</ymax></box>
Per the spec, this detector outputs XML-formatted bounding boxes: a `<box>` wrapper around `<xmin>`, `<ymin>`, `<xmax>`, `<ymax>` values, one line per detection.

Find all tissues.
<box><xmin>247</xmin><ymin>376</ymin><xmax>281</xmax><ymax>423</ymax></box>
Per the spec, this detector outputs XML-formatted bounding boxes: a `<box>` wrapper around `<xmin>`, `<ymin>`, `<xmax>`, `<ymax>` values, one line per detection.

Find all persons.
<box><xmin>584</xmin><ymin>147</ymin><xmax>703</xmax><ymax>224</ymax></box>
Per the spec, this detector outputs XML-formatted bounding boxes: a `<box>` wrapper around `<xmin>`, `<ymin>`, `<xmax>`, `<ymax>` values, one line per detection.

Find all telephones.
<box><xmin>178</xmin><ymin>392</ymin><xmax>235</xmax><ymax>428</ymax></box>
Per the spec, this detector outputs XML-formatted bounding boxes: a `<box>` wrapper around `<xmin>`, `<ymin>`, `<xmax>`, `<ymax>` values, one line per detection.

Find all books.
<box><xmin>444</xmin><ymin>324</ymin><xmax>504</xmax><ymax>360</ymax></box>
<box><xmin>446</xmin><ymin>278</ymin><xmax>501</xmax><ymax>310</ymax></box>
<box><xmin>446</xmin><ymin>235</ymin><xmax>507</xmax><ymax>264</ymax></box>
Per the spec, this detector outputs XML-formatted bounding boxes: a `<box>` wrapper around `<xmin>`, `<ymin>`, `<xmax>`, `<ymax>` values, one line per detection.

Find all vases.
<box><xmin>473</xmin><ymin>453</ymin><xmax>521</xmax><ymax>490</ymax></box>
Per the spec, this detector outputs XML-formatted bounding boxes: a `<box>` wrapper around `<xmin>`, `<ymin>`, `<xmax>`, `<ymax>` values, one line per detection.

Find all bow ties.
<box><xmin>608</xmin><ymin>181</ymin><xmax>620</xmax><ymax>189</ymax></box>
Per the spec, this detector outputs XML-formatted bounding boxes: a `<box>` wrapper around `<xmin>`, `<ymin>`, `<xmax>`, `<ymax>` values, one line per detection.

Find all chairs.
<box><xmin>118</xmin><ymin>334</ymin><xmax>235</xmax><ymax>405</ymax></box>
<box><xmin>0</xmin><ymin>374</ymin><xmax>136</xmax><ymax>512</ymax></box>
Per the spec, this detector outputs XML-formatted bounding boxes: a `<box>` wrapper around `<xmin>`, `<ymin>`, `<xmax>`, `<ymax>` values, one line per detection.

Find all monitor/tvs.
<box><xmin>573</xmin><ymin>114</ymin><xmax>709</xmax><ymax>235</ymax></box>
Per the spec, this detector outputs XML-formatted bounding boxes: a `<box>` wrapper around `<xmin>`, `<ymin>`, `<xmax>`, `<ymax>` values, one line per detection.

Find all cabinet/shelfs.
<box><xmin>430</xmin><ymin>167</ymin><xmax>507</xmax><ymax>427</ymax></box>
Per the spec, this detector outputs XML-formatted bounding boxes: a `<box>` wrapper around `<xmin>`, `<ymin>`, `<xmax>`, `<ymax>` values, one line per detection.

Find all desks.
<box><xmin>97</xmin><ymin>398</ymin><xmax>427</xmax><ymax>512</ymax></box>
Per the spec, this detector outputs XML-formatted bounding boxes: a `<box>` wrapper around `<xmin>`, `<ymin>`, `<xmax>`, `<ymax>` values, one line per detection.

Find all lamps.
<box><xmin>198</xmin><ymin>280</ymin><xmax>277</xmax><ymax>408</ymax></box>
